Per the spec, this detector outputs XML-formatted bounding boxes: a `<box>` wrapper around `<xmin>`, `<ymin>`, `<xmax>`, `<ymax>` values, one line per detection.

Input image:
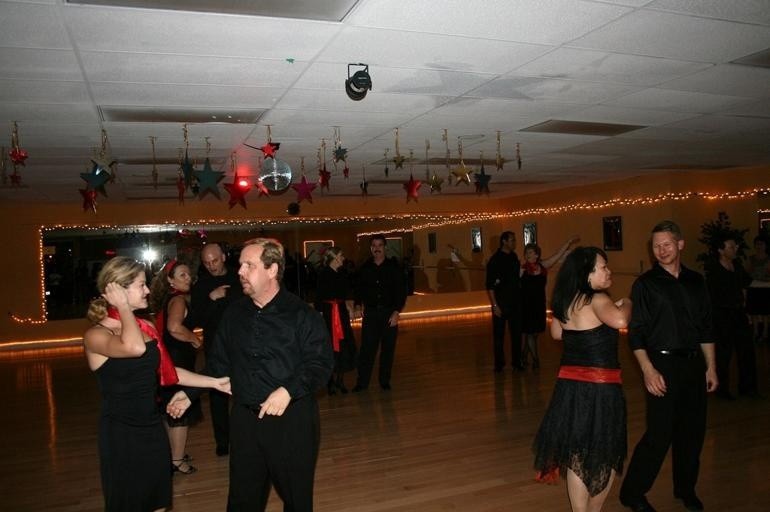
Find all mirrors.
<box><xmin>39</xmin><ymin>212</ymin><xmax>502</xmax><ymax>322</ymax></box>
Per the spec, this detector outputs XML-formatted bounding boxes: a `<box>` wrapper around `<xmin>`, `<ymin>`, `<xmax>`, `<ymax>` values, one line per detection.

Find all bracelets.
<box><xmin>566</xmin><ymin>239</ymin><xmax>572</xmax><ymax>244</ymax></box>
<box><xmin>493</xmin><ymin>303</ymin><xmax>498</xmax><ymax>307</ymax></box>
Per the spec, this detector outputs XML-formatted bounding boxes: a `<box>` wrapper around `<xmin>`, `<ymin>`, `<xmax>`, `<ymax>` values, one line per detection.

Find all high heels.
<box><xmin>183</xmin><ymin>455</ymin><xmax>195</xmax><ymax>461</ymax></box>
<box><xmin>328</xmin><ymin>380</ymin><xmax>336</xmax><ymax>397</ymax></box>
<box><xmin>335</xmin><ymin>381</ymin><xmax>348</xmax><ymax>394</ymax></box>
<box><xmin>171</xmin><ymin>458</ymin><xmax>198</xmax><ymax>477</ymax></box>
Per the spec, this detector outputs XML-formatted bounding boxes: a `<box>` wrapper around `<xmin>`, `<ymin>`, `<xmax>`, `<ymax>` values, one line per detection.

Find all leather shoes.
<box><xmin>352</xmin><ymin>384</ymin><xmax>368</xmax><ymax>393</ymax></box>
<box><xmin>216</xmin><ymin>440</ymin><xmax>229</xmax><ymax>456</ymax></box>
<box><xmin>683</xmin><ymin>495</ymin><xmax>704</xmax><ymax>512</ymax></box>
<box><xmin>631</xmin><ymin>496</ymin><xmax>656</xmax><ymax>512</ymax></box>
<box><xmin>381</xmin><ymin>383</ymin><xmax>390</xmax><ymax>391</ymax></box>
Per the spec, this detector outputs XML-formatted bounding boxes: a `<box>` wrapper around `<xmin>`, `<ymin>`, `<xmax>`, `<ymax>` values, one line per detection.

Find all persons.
<box><xmin>315</xmin><ymin>239</ymin><xmax>408</xmax><ymax>395</ymax></box>
<box><xmin>549</xmin><ymin>247</ymin><xmax>632</xmax><ymax>512</ymax></box>
<box><xmin>704</xmin><ymin>233</ymin><xmax>768</xmax><ymax>402</ymax></box>
<box><xmin>619</xmin><ymin>220</ymin><xmax>719</xmax><ymax>512</ymax></box>
<box><xmin>517</xmin><ymin>235</ymin><xmax>581</xmax><ymax>375</ymax></box>
<box><xmin>744</xmin><ymin>235</ymin><xmax>770</xmax><ymax>345</ymax></box>
<box><xmin>485</xmin><ymin>231</ymin><xmax>524</xmax><ymax>372</ymax></box>
<box><xmin>83</xmin><ymin>238</ymin><xmax>333</xmax><ymax>512</ymax></box>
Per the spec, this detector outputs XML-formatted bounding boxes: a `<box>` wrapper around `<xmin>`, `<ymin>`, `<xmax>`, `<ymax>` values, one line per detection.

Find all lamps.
<box><xmin>344</xmin><ymin>63</ymin><xmax>372</xmax><ymax>102</ymax></box>
